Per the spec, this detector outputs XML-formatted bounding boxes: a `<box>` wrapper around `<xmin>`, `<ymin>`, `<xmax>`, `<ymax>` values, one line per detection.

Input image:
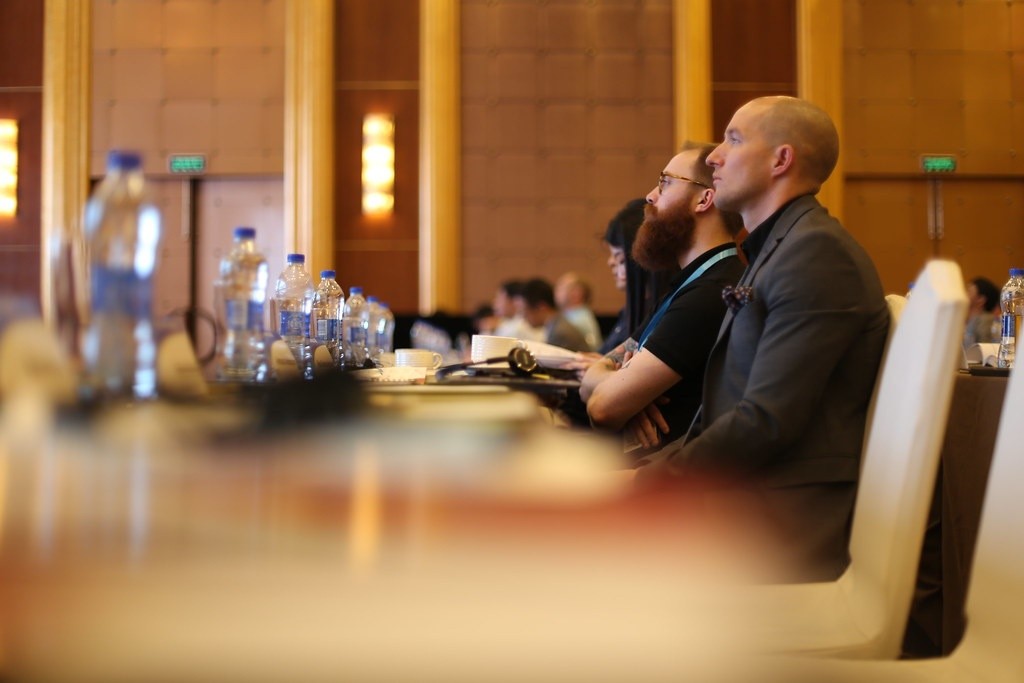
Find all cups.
<box><xmin>396</xmin><ymin>348</ymin><xmax>443</xmax><ymax>374</ymax></box>
<box><xmin>471</xmin><ymin>334</ymin><xmax>527</xmax><ymax>367</ymax></box>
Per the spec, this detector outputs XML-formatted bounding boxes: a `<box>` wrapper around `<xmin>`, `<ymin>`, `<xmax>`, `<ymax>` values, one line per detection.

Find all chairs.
<box><xmin>755</xmin><ymin>256</ymin><xmax>972</xmax><ymax>660</ymax></box>
<box><xmin>789</xmin><ymin>320</ymin><xmax>1022</xmax><ymax>683</ymax></box>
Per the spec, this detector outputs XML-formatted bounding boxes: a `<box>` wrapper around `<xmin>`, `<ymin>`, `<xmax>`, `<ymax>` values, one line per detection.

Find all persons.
<box><xmin>558</xmin><ymin>196</ymin><xmax>676</xmax><ymax>437</ymax></box>
<box><xmin>961</xmin><ymin>275</ymin><xmax>1003</xmax><ymax>351</ymax></box>
<box><xmin>574</xmin><ymin>138</ymin><xmax>747</xmax><ymax>470</ymax></box>
<box><xmin>613</xmin><ymin>94</ymin><xmax>892</xmax><ymax>586</ymax></box>
<box><xmin>434</xmin><ymin>267</ymin><xmax>604</xmax><ymax>375</ymax></box>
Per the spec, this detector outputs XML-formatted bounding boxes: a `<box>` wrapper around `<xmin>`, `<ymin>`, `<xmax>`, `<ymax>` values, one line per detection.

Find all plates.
<box><xmin>349</xmin><ymin>370</ymin><xmax>425</xmax><ymax>385</ymax></box>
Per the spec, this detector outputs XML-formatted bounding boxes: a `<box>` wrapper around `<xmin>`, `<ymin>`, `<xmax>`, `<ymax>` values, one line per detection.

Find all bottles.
<box><xmin>344</xmin><ymin>287</ymin><xmax>367</xmax><ymax>369</ymax></box>
<box><xmin>213</xmin><ymin>227</ymin><xmax>274</xmax><ymax>387</ymax></box>
<box><xmin>365</xmin><ymin>296</ymin><xmax>380</xmax><ymax>369</ymax></box>
<box><xmin>275</xmin><ymin>254</ymin><xmax>313</xmax><ymax>381</ymax></box>
<box><xmin>380</xmin><ymin>302</ymin><xmax>395</xmax><ymax>369</ymax></box>
<box><xmin>76</xmin><ymin>148</ymin><xmax>162</xmax><ymax>406</ymax></box>
<box><xmin>997</xmin><ymin>268</ymin><xmax>1023</xmax><ymax>369</ymax></box>
<box><xmin>314</xmin><ymin>270</ymin><xmax>345</xmax><ymax>380</ymax></box>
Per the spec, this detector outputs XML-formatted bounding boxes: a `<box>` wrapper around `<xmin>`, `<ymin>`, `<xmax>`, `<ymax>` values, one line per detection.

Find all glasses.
<box><xmin>658</xmin><ymin>174</ymin><xmax>710</xmax><ymax>191</ymax></box>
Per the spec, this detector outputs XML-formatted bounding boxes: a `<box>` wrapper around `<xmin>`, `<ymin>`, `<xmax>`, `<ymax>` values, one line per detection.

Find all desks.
<box><xmin>939</xmin><ymin>368</ymin><xmax>1024</xmax><ymax>683</ymax></box>
<box><xmin>1</xmin><ymin>351</ymin><xmax>789</xmax><ymax>683</ymax></box>
